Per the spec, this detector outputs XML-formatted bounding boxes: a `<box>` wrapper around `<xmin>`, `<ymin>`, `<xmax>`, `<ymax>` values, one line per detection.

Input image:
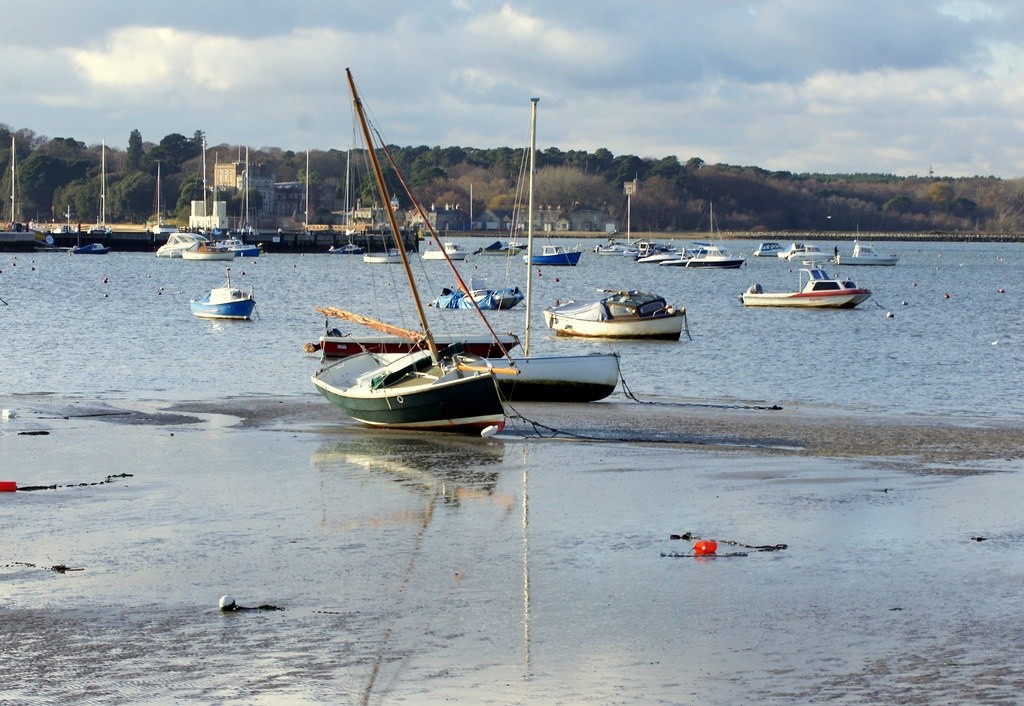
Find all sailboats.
<box><xmin>278</xmin><ymin>147</ymin><xmax>313</xmax><ymax>236</ymax></box>
<box><xmin>87</xmin><ymin>137</ymin><xmax>113</xmax><ymax>239</ymax></box>
<box><xmin>0</xmin><ymin>137</ymin><xmax>48</xmax><ymax>251</ymax></box>
<box><xmin>145</xmin><ymin>159</ymin><xmax>177</xmax><ymax>234</ymax></box>
<box><xmin>226</xmin><ymin>145</ymin><xmax>259</xmax><ymax>239</ymax></box>
<box><xmin>311</xmin><ymin>68</ymin><xmax>507</xmax><ymax>436</ymax></box>
<box><xmin>449</xmin><ymin>98</ymin><xmax>622</xmax><ymax>400</ymax></box>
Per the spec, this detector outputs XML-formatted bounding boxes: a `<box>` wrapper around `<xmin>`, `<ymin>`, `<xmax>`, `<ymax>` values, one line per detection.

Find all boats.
<box><xmin>432</xmin><ymin>287</ymin><xmax>525</xmax><ymax>310</ymax></box>
<box><xmin>189</xmin><ymin>267</ymin><xmax>256</xmax><ymax>319</ymax></box>
<box><xmin>737</xmin><ymin>268</ymin><xmax>873</xmax><ymax>308</ymax></box>
<box><xmin>69</xmin><ymin>243</ymin><xmax>110</xmax><ymax>254</ymax></box>
<box><xmin>181</xmin><ymin>241</ymin><xmax>235</xmax><ymax>261</ymax></box>
<box><xmin>314</xmin><ymin>171</ymin><xmax>900</xmax><ymax>269</ymax></box>
<box><xmin>53</xmin><ymin>205</ymin><xmax>76</xmax><ymax>233</ymax></box>
<box><xmin>318</xmin><ymin>336</ymin><xmax>520</xmax><ymax>360</ymax></box>
<box><xmin>542</xmin><ymin>290</ymin><xmax>685</xmax><ymax>340</ymax></box>
<box><xmin>156</xmin><ymin>232</ymin><xmax>208</xmax><ymax>257</ymax></box>
<box><xmin>216</xmin><ymin>237</ymin><xmax>262</xmax><ymax>257</ymax></box>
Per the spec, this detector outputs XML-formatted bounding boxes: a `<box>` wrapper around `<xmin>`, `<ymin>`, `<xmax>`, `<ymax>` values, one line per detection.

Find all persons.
<box><xmin>834</xmin><ymin>246</ymin><xmax>838</xmax><ymax>254</ymax></box>
